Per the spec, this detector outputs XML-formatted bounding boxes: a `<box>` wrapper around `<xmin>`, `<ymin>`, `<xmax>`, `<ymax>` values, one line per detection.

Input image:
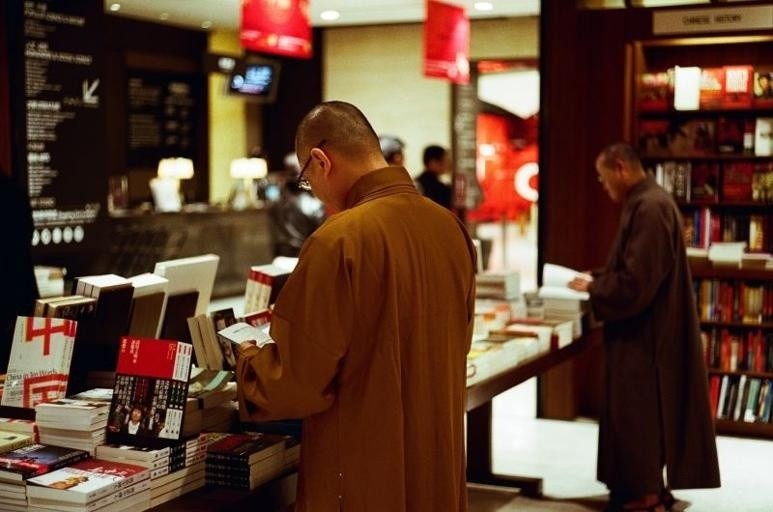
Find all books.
<box><xmin>1</xmin><ymin>316</ymin><xmax>77</xmax><ymax>410</ymax></box>
<box><xmin>648</xmin><ymin>162</ymin><xmax>773</xmax><ymax>201</ymax></box>
<box><xmin>709</xmin><ymin>375</ymin><xmax>773</xmax><ymax>424</ymax></box>
<box><xmin>642</xmin><ymin>65</ymin><xmax>773</xmax><ymax>110</ymax></box>
<box><xmin>0</xmin><ymin>389</ymin><xmax>112</xmax><ymax>433</ymax></box>
<box><xmin>0</xmin><ymin>433</ymin><xmax>302</xmax><ymax>512</ymax></box>
<box><xmin>682</xmin><ymin>207</ymin><xmax>773</xmax><ymax>271</ymax></box>
<box><xmin>640</xmin><ymin>118</ymin><xmax>773</xmax><ymax>156</ymax></box>
<box><xmin>212</xmin><ymin>254</ymin><xmax>296</xmax><ymax>386</ymax></box>
<box><xmin>693</xmin><ymin>276</ymin><xmax>773</xmax><ymax>323</ymax></box>
<box><xmin>34</xmin><ymin>295</ymin><xmax>68</xmax><ymax>319</ymax></box>
<box><xmin>701</xmin><ymin>328</ymin><xmax>773</xmax><ymax>373</ymax></box>
<box><xmin>467</xmin><ymin>263</ymin><xmax>595</xmax><ymax>385</ymax></box>
<box><xmin>183</xmin><ymin>371</ymin><xmax>239</xmax><ymax>435</ymax></box>
<box><xmin>106</xmin><ymin>335</ymin><xmax>191</xmax><ymax>441</ymax></box>
<box><xmin>68</xmin><ymin>253</ymin><xmax>222</xmax><ymax>337</ymax></box>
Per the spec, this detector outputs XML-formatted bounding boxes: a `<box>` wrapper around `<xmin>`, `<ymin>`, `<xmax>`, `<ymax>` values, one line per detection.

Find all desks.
<box><xmin>147</xmin><ymin>291</ymin><xmax>607</xmax><ymax>512</ymax></box>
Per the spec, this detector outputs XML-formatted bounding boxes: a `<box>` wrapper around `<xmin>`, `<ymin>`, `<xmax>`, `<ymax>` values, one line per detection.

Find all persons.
<box><xmin>238</xmin><ymin>102</ymin><xmax>475</xmax><ymax>512</ymax></box>
<box><xmin>415</xmin><ymin>146</ymin><xmax>452</xmax><ymax>211</ymax></box>
<box><xmin>379</xmin><ymin>137</ymin><xmax>404</xmax><ymax>167</ymax></box>
<box><xmin>571</xmin><ymin>146</ymin><xmax>720</xmax><ymax>512</ymax></box>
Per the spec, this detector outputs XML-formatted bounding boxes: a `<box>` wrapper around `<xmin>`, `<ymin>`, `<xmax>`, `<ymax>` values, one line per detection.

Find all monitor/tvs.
<box><xmin>228</xmin><ymin>61</ymin><xmax>274</xmax><ymax>98</ymax></box>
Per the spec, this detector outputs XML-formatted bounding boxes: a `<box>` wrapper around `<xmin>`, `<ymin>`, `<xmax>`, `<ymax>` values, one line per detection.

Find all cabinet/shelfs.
<box><xmin>632</xmin><ymin>35</ymin><xmax>773</xmax><ymax>439</ymax></box>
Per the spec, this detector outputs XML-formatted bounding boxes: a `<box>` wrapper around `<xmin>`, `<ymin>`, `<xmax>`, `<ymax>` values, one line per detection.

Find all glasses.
<box><xmin>295</xmin><ymin>140</ymin><xmax>330</xmax><ymax>191</ymax></box>
